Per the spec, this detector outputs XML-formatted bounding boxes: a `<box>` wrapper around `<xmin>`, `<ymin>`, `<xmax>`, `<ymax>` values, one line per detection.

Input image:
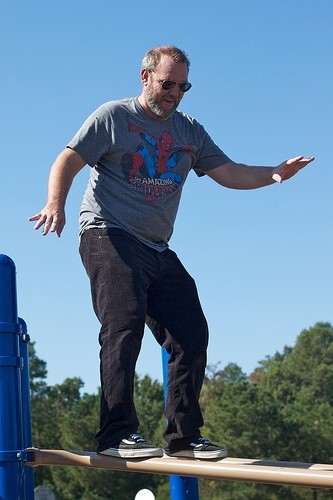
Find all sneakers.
<box><xmin>165</xmin><ymin>436</ymin><xmax>226</xmax><ymax>460</ymax></box>
<box><xmin>98</xmin><ymin>432</ymin><xmax>163</xmax><ymax>458</ymax></box>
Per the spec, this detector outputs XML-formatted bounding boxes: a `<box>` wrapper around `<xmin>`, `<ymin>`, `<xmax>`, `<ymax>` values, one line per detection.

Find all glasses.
<box><xmin>149</xmin><ymin>69</ymin><xmax>192</xmax><ymax>92</ymax></box>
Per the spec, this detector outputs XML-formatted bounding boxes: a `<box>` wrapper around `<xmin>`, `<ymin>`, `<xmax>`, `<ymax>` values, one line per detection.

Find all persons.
<box><xmin>26</xmin><ymin>46</ymin><xmax>317</xmax><ymax>461</ymax></box>
